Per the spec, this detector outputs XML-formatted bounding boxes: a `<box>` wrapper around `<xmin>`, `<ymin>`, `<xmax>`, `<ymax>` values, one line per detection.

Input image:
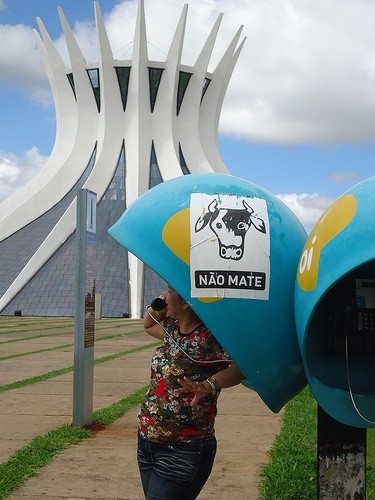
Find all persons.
<box><xmin>137</xmin><ymin>286</ymin><xmax>244</xmax><ymax>500</ymax></box>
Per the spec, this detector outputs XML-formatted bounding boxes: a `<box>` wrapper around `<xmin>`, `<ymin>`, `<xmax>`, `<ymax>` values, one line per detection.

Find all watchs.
<box><xmin>207</xmin><ymin>378</ymin><xmax>217</xmax><ymax>395</ymax></box>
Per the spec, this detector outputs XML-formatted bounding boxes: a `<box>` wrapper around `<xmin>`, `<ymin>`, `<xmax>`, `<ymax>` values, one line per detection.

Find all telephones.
<box><xmin>326</xmin><ymin>273</ymin><xmax>375</xmax><ymax>355</ymax></box>
<box><xmin>150</xmin><ymin>298</ymin><xmax>167</xmax><ymax>311</ymax></box>
